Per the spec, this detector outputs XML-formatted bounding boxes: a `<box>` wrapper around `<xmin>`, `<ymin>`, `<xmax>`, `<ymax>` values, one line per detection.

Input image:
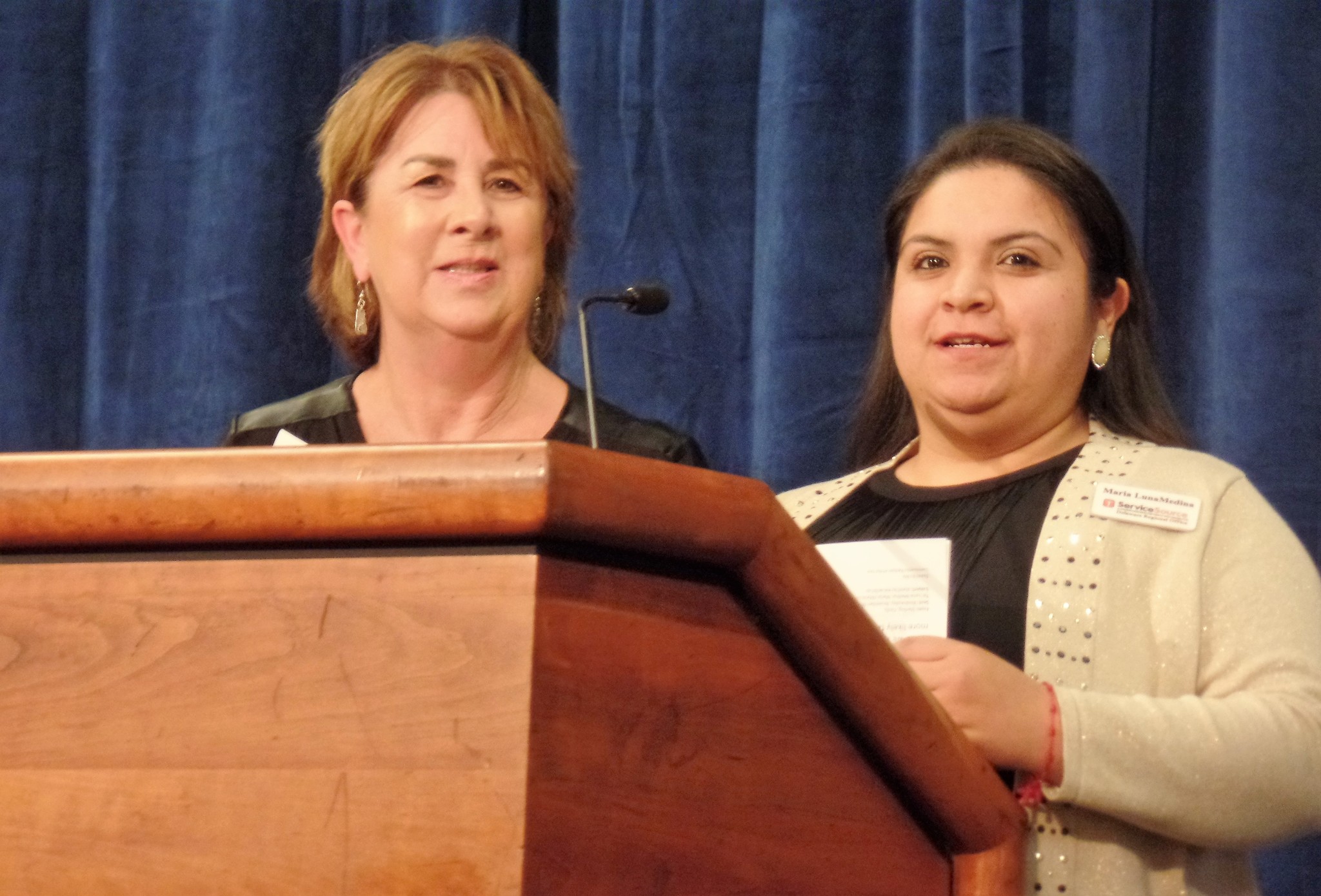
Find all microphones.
<box><xmin>578</xmin><ymin>282</ymin><xmax>675</xmax><ymax>450</ymax></box>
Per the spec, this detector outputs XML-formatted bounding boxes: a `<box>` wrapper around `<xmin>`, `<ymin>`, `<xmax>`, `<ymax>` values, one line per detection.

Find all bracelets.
<box><xmin>1016</xmin><ymin>681</ymin><xmax>1058</xmax><ymax>806</ymax></box>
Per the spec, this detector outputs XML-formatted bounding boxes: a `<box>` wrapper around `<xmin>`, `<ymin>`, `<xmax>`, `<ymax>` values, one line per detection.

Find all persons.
<box><xmin>774</xmin><ymin>117</ymin><xmax>1321</xmax><ymax>896</ymax></box>
<box><xmin>220</xmin><ymin>36</ymin><xmax>712</xmax><ymax>469</ymax></box>
